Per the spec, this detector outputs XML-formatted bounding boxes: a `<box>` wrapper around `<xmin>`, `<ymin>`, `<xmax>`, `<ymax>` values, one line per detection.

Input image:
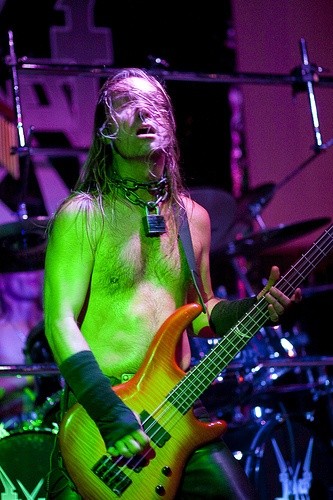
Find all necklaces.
<box><xmin>112</xmin><ymin>168</ymin><xmax>172</xmax><ymax>236</ymax></box>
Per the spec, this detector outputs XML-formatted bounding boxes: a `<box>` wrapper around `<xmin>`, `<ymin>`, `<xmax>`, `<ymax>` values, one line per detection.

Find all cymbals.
<box><xmin>0</xmin><ymin>216</ymin><xmax>53</xmax><ymax>275</ymax></box>
<box><xmin>216</xmin><ymin>215</ymin><xmax>332</xmax><ymax>259</ymax></box>
<box><xmin>189</xmin><ymin>190</ymin><xmax>237</xmax><ymax>246</ymax></box>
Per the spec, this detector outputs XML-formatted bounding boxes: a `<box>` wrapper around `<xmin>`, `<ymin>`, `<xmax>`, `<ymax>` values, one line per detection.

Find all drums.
<box><xmin>0</xmin><ymin>417</ymin><xmax>62</xmax><ymax>500</ymax></box>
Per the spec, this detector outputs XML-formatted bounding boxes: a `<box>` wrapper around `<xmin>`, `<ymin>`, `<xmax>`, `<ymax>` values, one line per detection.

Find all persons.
<box><xmin>41</xmin><ymin>66</ymin><xmax>301</xmax><ymax>500</ymax></box>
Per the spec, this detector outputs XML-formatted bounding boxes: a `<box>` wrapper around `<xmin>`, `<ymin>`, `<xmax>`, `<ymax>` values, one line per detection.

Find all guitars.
<box><xmin>59</xmin><ymin>226</ymin><xmax>333</xmax><ymax>500</ymax></box>
